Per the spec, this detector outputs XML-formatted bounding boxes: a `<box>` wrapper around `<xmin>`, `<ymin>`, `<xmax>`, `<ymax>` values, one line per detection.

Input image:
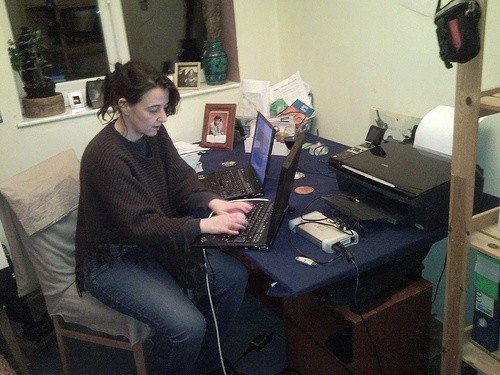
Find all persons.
<box><xmin>75</xmin><ymin>60</ymin><xmax>255</xmax><ymax>375</ymax></box>
<box><xmin>210</xmin><ymin>115</ymin><xmax>224</xmax><ymax>135</ymax></box>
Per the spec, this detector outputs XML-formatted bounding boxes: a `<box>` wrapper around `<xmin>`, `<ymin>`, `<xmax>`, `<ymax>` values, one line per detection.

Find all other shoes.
<box><xmin>207</xmin><ymin>334</ymin><xmax>221</xmax><ymax>360</ymax></box>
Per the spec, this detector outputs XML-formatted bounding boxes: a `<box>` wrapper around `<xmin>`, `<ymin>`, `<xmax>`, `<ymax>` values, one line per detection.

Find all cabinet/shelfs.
<box><xmin>438</xmin><ymin>0</ymin><xmax>500</xmax><ymax>375</ymax></box>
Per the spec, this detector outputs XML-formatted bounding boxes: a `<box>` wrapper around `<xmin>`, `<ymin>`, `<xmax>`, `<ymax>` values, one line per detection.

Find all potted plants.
<box><xmin>7</xmin><ymin>25</ymin><xmax>56</xmax><ymax>98</ymax></box>
<box><xmin>200</xmin><ymin>0</ymin><xmax>228</xmax><ymax>86</ymax></box>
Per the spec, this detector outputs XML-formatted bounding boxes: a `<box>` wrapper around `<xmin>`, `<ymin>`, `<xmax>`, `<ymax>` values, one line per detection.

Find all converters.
<box><xmin>251</xmin><ymin>327</ymin><xmax>273</xmax><ymax>352</ymax></box>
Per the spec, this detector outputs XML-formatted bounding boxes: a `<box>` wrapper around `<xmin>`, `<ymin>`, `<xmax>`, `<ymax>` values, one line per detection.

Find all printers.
<box><xmin>318</xmin><ymin>113</ymin><xmax>486</xmax><ymax>231</ymax></box>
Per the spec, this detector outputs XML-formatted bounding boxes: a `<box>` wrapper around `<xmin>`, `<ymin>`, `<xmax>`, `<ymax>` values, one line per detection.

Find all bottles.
<box><xmin>179</xmin><ymin>38</ymin><xmax>200</xmax><ymax>62</ymax></box>
<box><xmin>202</xmin><ymin>41</ymin><xmax>228</xmax><ymax>85</ymax></box>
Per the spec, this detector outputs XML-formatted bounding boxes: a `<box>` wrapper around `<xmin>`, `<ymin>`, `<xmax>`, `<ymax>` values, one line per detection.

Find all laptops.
<box><xmin>195</xmin><ymin>110</ymin><xmax>275</xmax><ymax>203</ymax></box>
<box><xmin>193</xmin><ymin>131</ymin><xmax>304</xmax><ymax>249</ymax></box>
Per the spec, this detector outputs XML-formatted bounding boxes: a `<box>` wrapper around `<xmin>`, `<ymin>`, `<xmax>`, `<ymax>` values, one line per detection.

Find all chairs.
<box><xmin>0</xmin><ymin>149</ymin><xmax>193</xmax><ymax>375</ymax></box>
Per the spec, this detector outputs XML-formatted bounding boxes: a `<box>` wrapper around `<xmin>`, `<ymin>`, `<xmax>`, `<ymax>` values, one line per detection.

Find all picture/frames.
<box><xmin>201</xmin><ymin>103</ymin><xmax>237</xmax><ymax>151</ymax></box>
<box><xmin>174</xmin><ymin>61</ymin><xmax>201</xmax><ymax>90</ymax></box>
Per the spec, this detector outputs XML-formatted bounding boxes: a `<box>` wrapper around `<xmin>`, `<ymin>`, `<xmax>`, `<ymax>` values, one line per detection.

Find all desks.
<box><xmin>191</xmin><ymin>131</ymin><xmax>500</xmax><ymax>375</ymax></box>
